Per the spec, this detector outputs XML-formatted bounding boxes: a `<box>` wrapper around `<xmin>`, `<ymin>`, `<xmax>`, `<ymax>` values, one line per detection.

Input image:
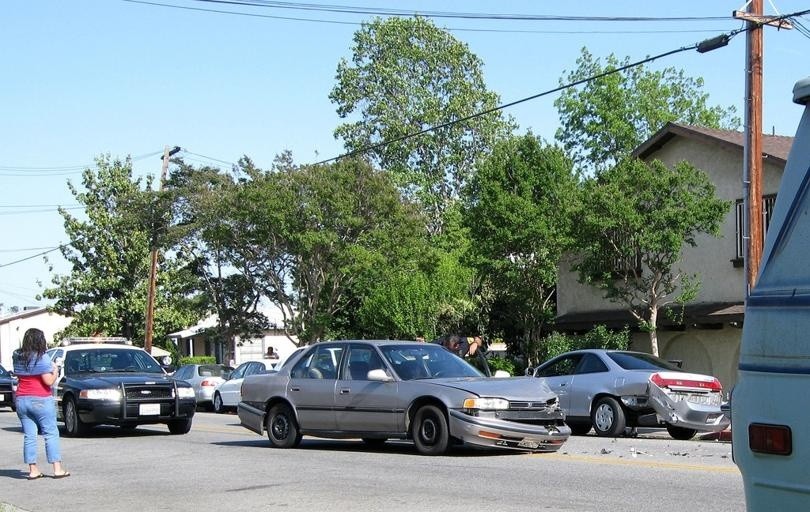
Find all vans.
<box><xmin>729</xmin><ymin>80</ymin><xmax>809</xmax><ymax>512</ymax></box>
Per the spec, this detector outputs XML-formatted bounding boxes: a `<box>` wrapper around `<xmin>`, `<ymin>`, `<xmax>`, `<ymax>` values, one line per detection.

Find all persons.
<box><xmin>10</xmin><ymin>328</ymin><xmax>70</xmax><ymax>481</ymax></box>
<box><xmin>262</xmin><ymin>346</ymin><xmax>280</xmax><ymax>369</ymax></box>
<box><xmin>430</xmin><ymin>333</ymin><xmax>484</xmax><ymax>357</ymax></box>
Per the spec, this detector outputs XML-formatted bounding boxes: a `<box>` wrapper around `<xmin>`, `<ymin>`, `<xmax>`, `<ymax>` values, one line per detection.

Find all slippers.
<box><xmin>28</xmin><ymin>472</ymin><xmax>44</xmax><ymax>480</ymax></box>
<box><xmin>52</xmin><ymin>470</ymin><xmax>70</xmax><ymax>479</ymax></box>
